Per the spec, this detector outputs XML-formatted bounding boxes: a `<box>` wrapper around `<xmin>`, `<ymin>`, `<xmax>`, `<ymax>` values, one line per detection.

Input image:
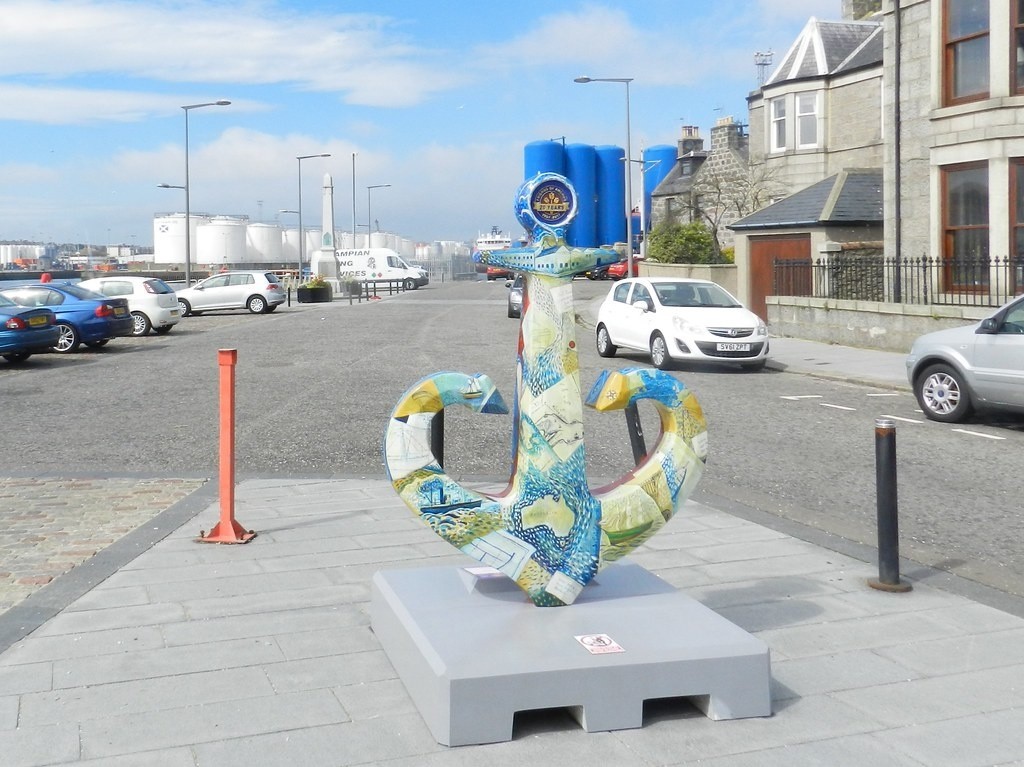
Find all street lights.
<box><xmin>573</xmin><ymin>76</ymin><xmax>647</xmax><ymax>278</ymax></box>
<box><xmin>280</xmin><ymin>153</ymin><xmax>331</xmax><ymax>286</ymax></box>
<box><xmin>157</xmin><ymin>100</ymin><xmax>232</xmax><ymax>286</ymax></box>
<box><xmin>357</xmin><ymin>184</ymin><xmax>390</xmax><ymax>247</ymax></box>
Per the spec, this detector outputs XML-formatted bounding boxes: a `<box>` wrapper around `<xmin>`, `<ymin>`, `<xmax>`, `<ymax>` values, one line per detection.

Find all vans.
<box><xmin>335</xmin><ymin>248</ymin><xmax>429</xmax><ymax>290</ymax></box>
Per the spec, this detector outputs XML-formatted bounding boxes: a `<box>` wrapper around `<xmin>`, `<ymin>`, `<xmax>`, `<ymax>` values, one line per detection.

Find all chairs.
<box><xmin>641</xmin><ymin>287</ymin><xmax>654</xmax><ymax>310</ymax></box>
<box><xmin>679</xmin><ymin>286</ymin><xmax>699</xmax><ymax>305</ymax></box>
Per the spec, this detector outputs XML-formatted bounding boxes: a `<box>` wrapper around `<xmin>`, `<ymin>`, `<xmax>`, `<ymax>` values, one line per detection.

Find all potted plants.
<box><xmin>297</xmin><ymin>273</ymin><xmax>333</xmax><ymax>303</ymax></box>
<box><xmin>337</xmin><ymin>273</ymin><xmax>362</xmax><ymax>296</ymax></box>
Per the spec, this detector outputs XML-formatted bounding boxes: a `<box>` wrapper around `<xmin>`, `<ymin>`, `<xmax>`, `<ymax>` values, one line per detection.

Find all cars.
<box><xmin>585</xmin><ymin>260</ymin><xmax>618</xmax><ymax>280</ymax></box>
<box><xmin>77</xmin><ymin>276</ymin><xmax>182</xmax><ymax>337</ymax></box>
<box><xmin>0</xmin><ymin>292</ymin><xmax>60</xmax><ymax>362</ymax></box>
<box><xmin>487</xmin><ymin>263</ymin><xmax>514</xmax><ymax>281</ymax></box>
<box><xmin>595</xmin><ymin>276</ymin><xmax>769</xmax><ymax>370</ymax></box>
<box><xmin>505</xmin><ymin>275</ymin><xmax>524</xmax><ymax>318</ymax></box>
<box><xmin>0</xmin><ymin>283</ymin><xmax>134</xmax><ymax>354</ymax></box>
<box><xmin>607</xmin><ymin>257</ymin><xmax>646</xmax><ymax>280</ymax></box>
<box><xmin>174</xmin><ymin>271</ymin><xmax>286</xmax><ymax>317</ymax></box>
<box><xmin>906</xmin><ymin>294</ymin><xmax>1024</xmax><ymax>422</ymax></box>
